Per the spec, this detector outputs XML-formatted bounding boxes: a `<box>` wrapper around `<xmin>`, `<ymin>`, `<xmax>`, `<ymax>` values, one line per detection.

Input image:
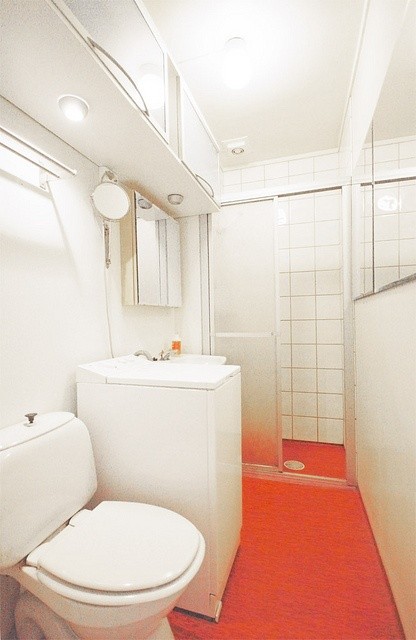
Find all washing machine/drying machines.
<box><xmin>76</xmin><ymin>354</ymin><xmax>243</xmax><ymax>623</ymax></box>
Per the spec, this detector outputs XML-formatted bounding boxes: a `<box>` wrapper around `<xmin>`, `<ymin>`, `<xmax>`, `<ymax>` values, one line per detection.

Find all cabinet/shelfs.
<box><xmin>120</xmin><ymin>187</ymin><xmax>182</xmax><ymax>308</ymax></box>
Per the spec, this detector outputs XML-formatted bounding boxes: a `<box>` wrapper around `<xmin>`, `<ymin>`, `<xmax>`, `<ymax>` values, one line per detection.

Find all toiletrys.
<box><xmin>171</xmin><ymin>334</ymin><xmax>183</xmax><ymax>355</ymax></box>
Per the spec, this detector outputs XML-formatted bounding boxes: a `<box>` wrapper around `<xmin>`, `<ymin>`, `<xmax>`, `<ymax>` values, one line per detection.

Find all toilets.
<box><xmin>0</xmin><ymin>406</ymin><xmax>206</xmax><ymax>640</ymax></box>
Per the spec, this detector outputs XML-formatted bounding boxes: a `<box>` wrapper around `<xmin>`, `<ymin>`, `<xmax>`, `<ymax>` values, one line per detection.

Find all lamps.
<box><xmin>168</xmin><ymin>195</ymin><xmax>184</xmax><ymax>205</ymax></box>
<box><xmin>93</xmin><ymin>170</ymin><xmax>131</xmax><ymax>220</ymax></box>
